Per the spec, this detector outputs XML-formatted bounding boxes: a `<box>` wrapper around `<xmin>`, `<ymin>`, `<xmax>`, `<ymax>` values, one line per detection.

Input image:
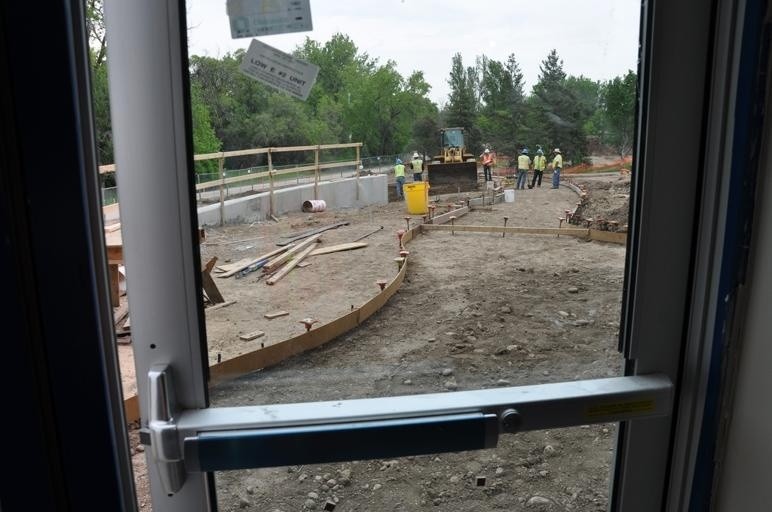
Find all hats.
<box><xmin>412</xmin><ymin>153</ymin><xmax>419</xmax><ymax>157</ymax></box>
<box><xmin>553</xmin><ymin>148</ymin><xmax>562</xmax><ymax>153</ymax></box>
<box><xmin>396</xmin><ymin>159</ymin><xmax>402</xmax><ymax>164</ymax></box>
<box><xmin>484</xmin><ymin>149</ymin><xmax>489</xmax><ymax>153</ymax></box>
<box><xmin>537</xmin><ymin>149</ymin><xmax>543</xmax><ymax>153</ymax></box>
<box><xmin>522</xmin><ymin>149</ymin><xmax>528</xmax><ymax>153</ymax></box>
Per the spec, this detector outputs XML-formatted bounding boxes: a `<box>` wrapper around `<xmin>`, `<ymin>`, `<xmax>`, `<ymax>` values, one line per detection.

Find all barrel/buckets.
<box><xmin>303</xmin><ymin>199</ymin><xmax>327</xmax><ymax>213</ymax></box>
<box><xmin>487</xmin><ymin>181</ymin><xmax>494</xmax><ymax>189</ymax></box>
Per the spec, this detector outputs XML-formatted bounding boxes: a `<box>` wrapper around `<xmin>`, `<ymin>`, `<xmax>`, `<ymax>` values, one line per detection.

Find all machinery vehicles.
<box><xmin>427</xmin><ymin>126</ymin><xmax>477</xmax><ymax>194</ymax></box>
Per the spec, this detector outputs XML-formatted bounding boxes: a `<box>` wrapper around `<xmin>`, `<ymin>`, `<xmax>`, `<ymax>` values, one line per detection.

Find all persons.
<box><xmin>393</xmin><ymin>158</ymin><xmax>406</xmax><ymax>200</ymax></box>
<box><xmin>516</xmin><ymin>149</ymin><xmax>532</xmax><ymax>189</ymax></box>
<box><xmin>549</xmin><ymin>148</ymin><xmax>562</xmax><ymax>189</ymax></box>
<box><xmin>410</xmin><ymin>152</ymin><xmax>424</xmax><ymax>182</ymax></box>
<box><xmin>531</xmin><ymin>149</ymin><xmax>547</xmax><ymax>188</ymax></box>
<box><xmin>480</xmin><ymin>145</ymin><xmax>496</xmax><ymax>183</ymax></box>
